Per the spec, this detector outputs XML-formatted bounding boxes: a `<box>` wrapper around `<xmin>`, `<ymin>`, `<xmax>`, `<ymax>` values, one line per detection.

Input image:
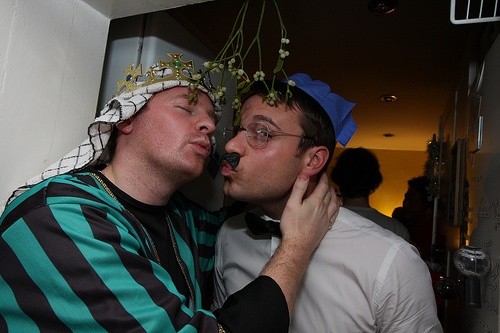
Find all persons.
<box><xmin>392</xmin><ymin>177</ymin><xmax>442</xmax><ymax>261</ymax></box>
<box><xmin>213</xmin><ymin>73</ymin><xmax>442</xmax><ymax>333</ymax></box>
<box><xmin>0</xmin><ymin>52</ymin><xmax>343</xmax><ymax>333</ymax></box>
<box><xmin>333</xmin><ymin>148</ymin><xmax>411</xmax><ymax>243</ymax></box>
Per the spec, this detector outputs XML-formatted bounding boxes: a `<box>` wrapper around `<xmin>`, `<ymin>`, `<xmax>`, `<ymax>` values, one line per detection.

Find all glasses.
<box><xmin>222</xmin><ymin>121</ymin><xmax>314</xmax><ymax>149</ymax></box>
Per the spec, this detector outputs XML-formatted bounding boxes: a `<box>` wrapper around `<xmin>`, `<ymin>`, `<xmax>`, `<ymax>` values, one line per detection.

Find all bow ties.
<box><xmin>244</xmin><ymin>213</ymin><xmax>283</xmax><ymax>240</ymax></box>
